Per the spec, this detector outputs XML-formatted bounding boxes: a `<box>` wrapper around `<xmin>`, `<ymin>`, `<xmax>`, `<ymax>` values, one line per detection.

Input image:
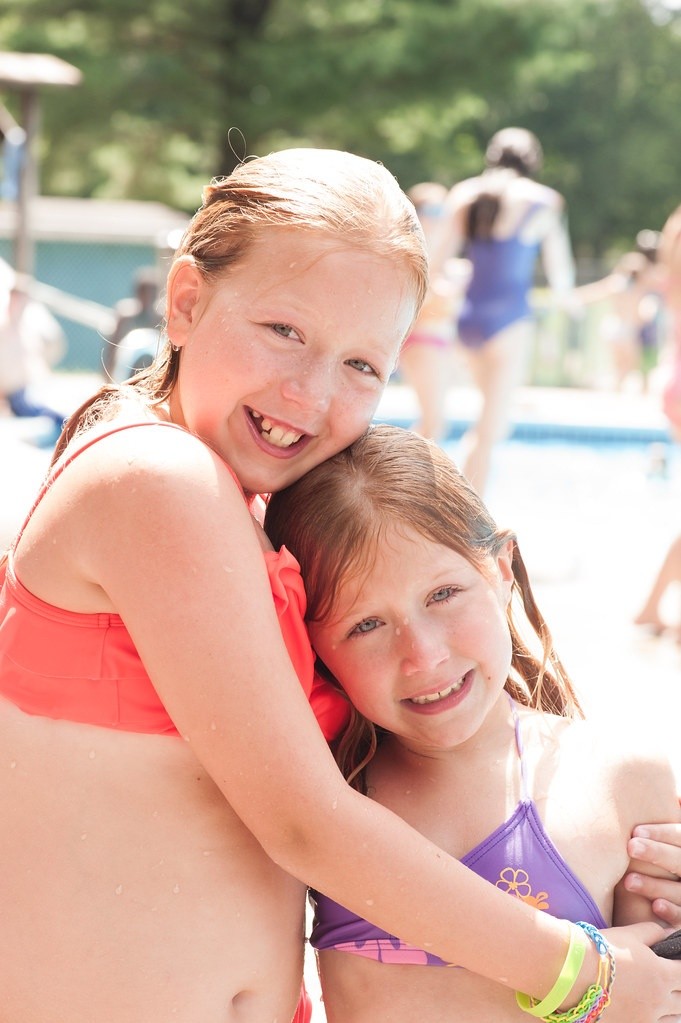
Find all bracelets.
<box><xmin>514</xmin><ymin>915</ymin><xmax>615</xmax><ymax>1023</ymax></box>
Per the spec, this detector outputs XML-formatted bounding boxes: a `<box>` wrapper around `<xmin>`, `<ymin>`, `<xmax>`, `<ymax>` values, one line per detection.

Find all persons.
<box><xmin>0</xmin><ymin>136</ymin><xmax>681</xmax><ymax>1023</ymax></box>
<box><xmin>0</xmin><ymin>122</ymin><xmax>681</xmax><ymax>638</ymax></box>
<box><xmin>263</xmin><ymin>421</ymin><xmax>681</xmax><ymax>1023</ymax></box>
